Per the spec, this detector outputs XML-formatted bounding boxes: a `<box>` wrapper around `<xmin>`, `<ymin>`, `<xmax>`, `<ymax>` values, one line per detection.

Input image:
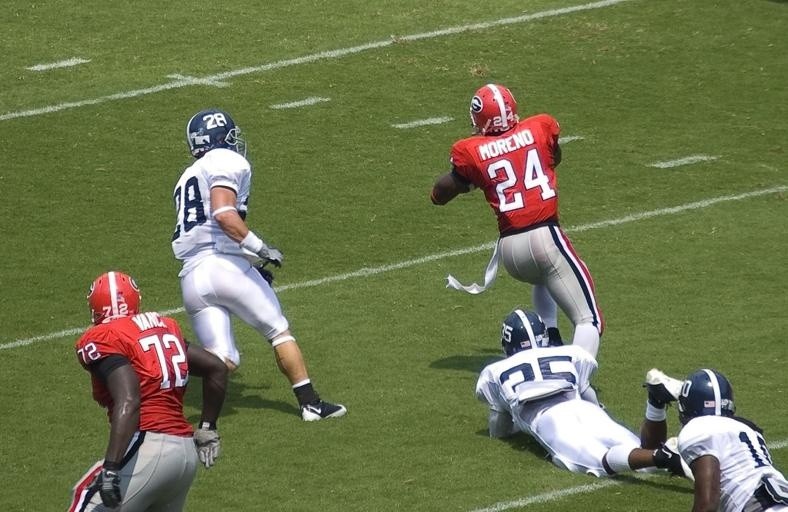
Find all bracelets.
<box><xmin>239</xmin><ymin>230</ymin><xmax>263</xmax><ymax>254</ymax></box>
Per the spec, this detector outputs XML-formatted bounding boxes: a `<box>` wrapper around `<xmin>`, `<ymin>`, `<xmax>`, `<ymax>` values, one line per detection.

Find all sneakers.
<box><xmin>547</xmin><ymin>327</ymin><xmax>563</xmax><ymax>346</ymax></box>
<box><xmin>659</xmin><ymin>436</ymin><xmax>695</xmax><ymax>486</ymax></box>
<box><xmin>299</xmin><ymin>390</ymin><xmax>348</xmax><ymax>422</ymax></box>
<box><xmin>641</xmin><ymin>368</ymin><xmax>684</xmax><ymax>412</ymax></box>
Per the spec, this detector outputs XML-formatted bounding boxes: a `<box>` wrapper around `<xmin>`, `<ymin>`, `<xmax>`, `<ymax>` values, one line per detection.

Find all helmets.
<box><xmin>186</xmin><ymin>108</ymin><xmax>247</xmax><ymax>157</ymax></box>
<box><xmin>87</xmin><ymin>271</ymin><xmax>142</xmax><ymax>325</ymax></box>
<box><xmin>501</xmin><ymin>309</ymin><xmax>550</xmax><ymax>356</ymax></box>
<box><xmin>469</xmin><ymin>83</ymin><xmax>520</xmax><ymax>137</ymax></box>
<box><xmin>679</xmin><ymin>368</ymin><xmax>737</xmax><ymax>427</ymax></box>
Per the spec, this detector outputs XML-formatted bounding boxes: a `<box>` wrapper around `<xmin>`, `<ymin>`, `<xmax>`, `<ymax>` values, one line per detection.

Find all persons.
<box><xmin>475</xmin><ymin>307</ymin><xmax>695</xmax><ymax>483</ymax></box>
<box><xmin>170</xmin><ymin>108</ymin><xmax>345</xmax><ymax>422</ymax></box>
<box><xmin>676</xmin><ymin>368</ymin><xmax>788</xmax><ymax>512</ymax></box>
<box><xmin>66</xmin><ymin>271</ymin><xmax>229</xmax><ymax>512</ymax></box>
<box><xmin>430</xmin><ymin>83</ymin><xmax>604</xmax><ymax>359</ymax></box>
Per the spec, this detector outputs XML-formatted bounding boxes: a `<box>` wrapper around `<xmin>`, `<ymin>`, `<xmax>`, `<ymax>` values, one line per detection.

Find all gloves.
<box><xmin>253</xmin><ymin>265</ymin><xmax>275</xmax><ymax>286</ymax></box>
<box><xmin>257</xmin><ymin>240</ymin><xmax>284</xmax><ymax>269</ymax></box>
<box><xmin>193</xmin><ymin>421</ymin><xmax>220</xmax><ymax>469</ymax></box>
<box><xmin>87</xmin><ymin>462</ymin><xmax>122</xmax><ymax>509</ymax></box>
